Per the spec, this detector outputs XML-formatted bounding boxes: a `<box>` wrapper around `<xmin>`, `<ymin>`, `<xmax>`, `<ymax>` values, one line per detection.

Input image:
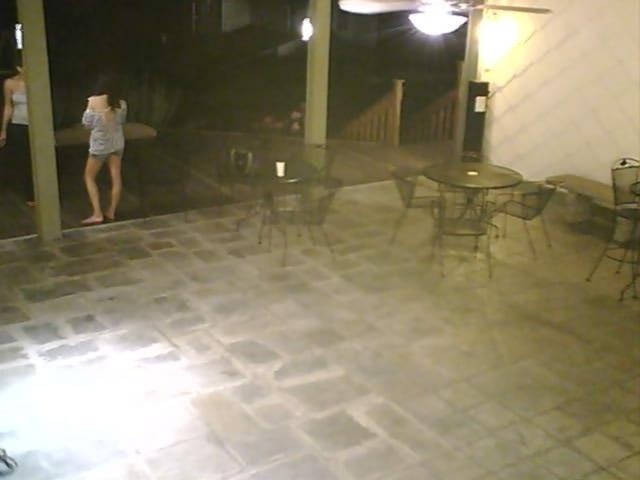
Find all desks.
<box><xmin>236</xmin><ymin>159</ymin><xmax>320</xmax><ymax>246</ymax></box>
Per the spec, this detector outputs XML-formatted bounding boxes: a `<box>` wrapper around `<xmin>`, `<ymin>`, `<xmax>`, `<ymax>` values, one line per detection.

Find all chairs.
<box><xmin>585</xmin><ymin>165</ymin><xmax>640</xmax><ymax>300</ymax></box>
<box><xmin>392</xmin><ymin>152</ymin><xmax>556</xmax><ymax>279</ymax></box>
<box><xmin>259</xmin><ymin>142</ymin><xmax>339</xmax><ymax>258</ymax></box>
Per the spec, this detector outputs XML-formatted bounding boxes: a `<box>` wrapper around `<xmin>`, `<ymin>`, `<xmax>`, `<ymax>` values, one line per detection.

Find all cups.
<box><xmin>276</xmin><ymin>162</ymin><xmax>286</xmax><ymax>176</ymax></box>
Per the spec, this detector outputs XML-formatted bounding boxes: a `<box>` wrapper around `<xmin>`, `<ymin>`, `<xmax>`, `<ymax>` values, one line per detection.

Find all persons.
<box><xmin>0</xmin><ymin>56</ymin><xmax>36</xmax><ymax>208</ymax></box>
<box><xmin>79</xmin><ymin>78</ymin><xmax>129</xmax><ymax>225</ymax></box>
